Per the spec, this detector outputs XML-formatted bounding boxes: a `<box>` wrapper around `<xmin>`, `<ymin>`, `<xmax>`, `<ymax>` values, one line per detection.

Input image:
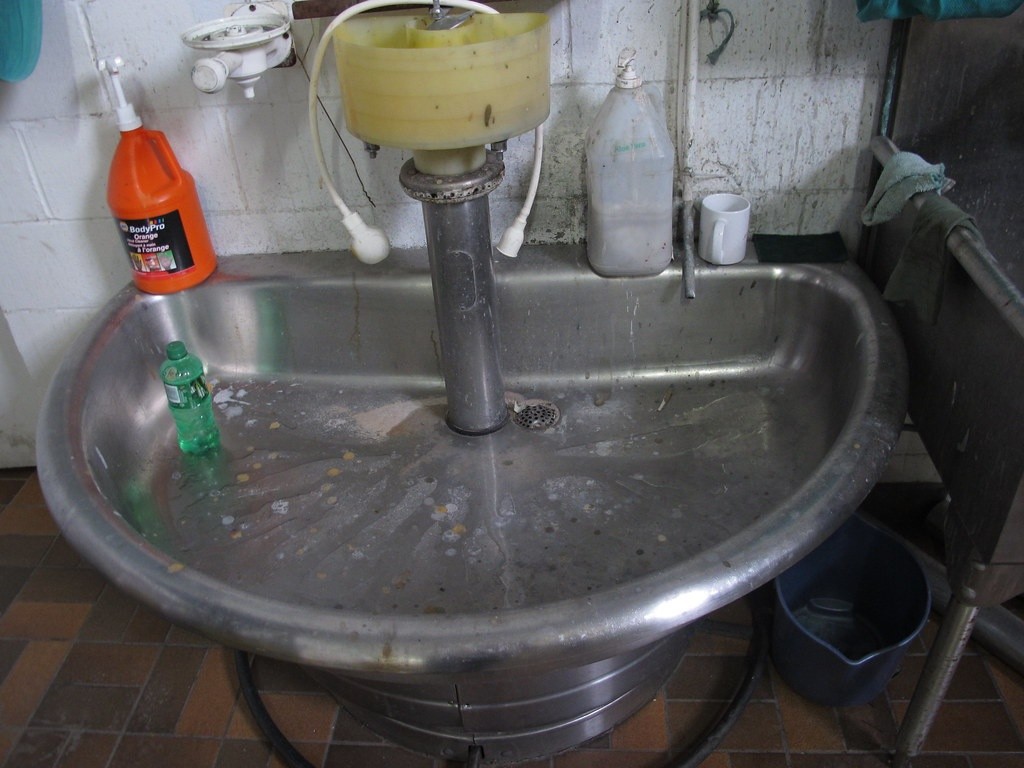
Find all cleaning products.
<box><xmin>94</xmin><ymin>53</ymin><xmax>217</xmax><ymax>295</ymax></box>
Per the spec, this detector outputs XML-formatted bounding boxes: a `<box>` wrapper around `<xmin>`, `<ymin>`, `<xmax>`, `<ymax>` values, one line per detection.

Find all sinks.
<box><xmin>33</xmin><ymin>248</ymin><xmax>914</xmax><ymax>680</ymax></box>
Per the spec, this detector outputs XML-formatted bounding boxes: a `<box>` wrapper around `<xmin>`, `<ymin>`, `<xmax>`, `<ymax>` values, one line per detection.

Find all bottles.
<box><xmin>161</xmin><ymin>343</ymin><xmax>217</xmax><ymax>456</ymax></box>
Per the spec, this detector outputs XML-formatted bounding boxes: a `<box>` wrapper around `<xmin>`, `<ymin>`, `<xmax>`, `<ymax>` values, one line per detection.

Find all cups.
<box><xmin>700</xmin><ymin>195</ymin><xmax>754</xmax><ymax>267</ymax></box>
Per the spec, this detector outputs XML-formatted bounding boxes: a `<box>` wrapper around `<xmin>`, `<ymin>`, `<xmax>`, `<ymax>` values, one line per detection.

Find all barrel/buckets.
<box><xmin>773</xmin><ymin>510</ymin><xmax>931</xmax><ymax>713</ymax></box>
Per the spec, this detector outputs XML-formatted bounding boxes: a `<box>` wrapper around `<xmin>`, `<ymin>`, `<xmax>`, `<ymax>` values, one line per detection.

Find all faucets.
<box><xmin>180</xmin><ymin>14</ymin><xmax>293</xmax><ymax>99</ymax></box>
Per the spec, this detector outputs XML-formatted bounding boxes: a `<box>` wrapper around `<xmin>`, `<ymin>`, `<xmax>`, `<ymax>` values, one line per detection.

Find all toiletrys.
<box><xmin>584</xmin><ymin>49</ymin><xmax>677</xmax><ymax>277</ymax></box>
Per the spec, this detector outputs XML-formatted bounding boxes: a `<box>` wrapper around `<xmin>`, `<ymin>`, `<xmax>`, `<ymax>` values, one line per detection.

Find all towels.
<box><xmin>861</xmin><ymin>151</ymin><xmax>989</xmax><ymax>340</ymax></box>
<box><xmin>855</xmin><ymin>0</ymin><xmax>1024</xmax><ymax>22</ymax></box>
